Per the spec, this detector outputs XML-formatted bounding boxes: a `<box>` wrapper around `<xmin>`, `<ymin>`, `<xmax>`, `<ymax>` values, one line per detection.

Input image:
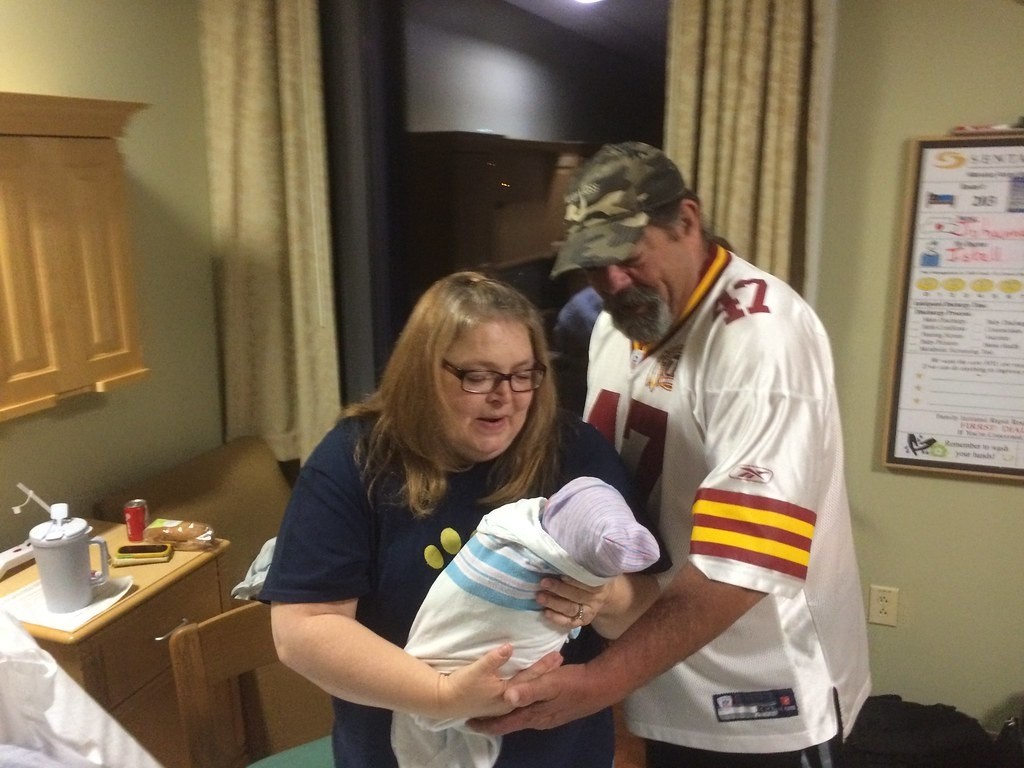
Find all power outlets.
<box><xmin>868</xmin><ymin>584</ymin><xmax>900</xmax><ymax>627</ymax></box>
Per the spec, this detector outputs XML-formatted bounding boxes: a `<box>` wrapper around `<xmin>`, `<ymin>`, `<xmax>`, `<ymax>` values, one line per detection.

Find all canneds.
<box><xmin>123</xmin><ymin>498</ymin><xmax>150</xmax><ymax>542</ymax></box>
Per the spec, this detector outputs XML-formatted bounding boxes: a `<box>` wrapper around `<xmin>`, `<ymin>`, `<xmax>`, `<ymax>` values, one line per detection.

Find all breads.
<box><xmin>143</xmin><ymin>518</ymin><xmax>214</xmax><ymax>550</ymax></box>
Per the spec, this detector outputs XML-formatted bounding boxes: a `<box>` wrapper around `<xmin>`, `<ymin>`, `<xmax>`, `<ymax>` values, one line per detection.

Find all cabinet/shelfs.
<box><xmin>0</xmin><ymin>518</ymin><xmax>252</xmax><ymax>768</ymax></box>
<box><xmin>0</xmin><ymin>91</ymin><xmax>149</xmax><ymax>423</ymax></box>
<box><xmin>406</xmin><ymin>130</ymin><xmax>603</xmax><ymax>311</ymax></box>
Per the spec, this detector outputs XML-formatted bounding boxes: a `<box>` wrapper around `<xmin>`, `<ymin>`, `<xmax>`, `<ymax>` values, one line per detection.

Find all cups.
<box><xmin>29</xmin><ymin>503</ymin><xmax>109</xmax><ymax>612</ymax></box>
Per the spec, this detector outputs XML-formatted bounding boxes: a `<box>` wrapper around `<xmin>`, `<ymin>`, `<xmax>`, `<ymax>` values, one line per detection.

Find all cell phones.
<box><xmin>116</xmin><ymin>543</ymin><xmax>171</xmax><ymax>558</ymax></box>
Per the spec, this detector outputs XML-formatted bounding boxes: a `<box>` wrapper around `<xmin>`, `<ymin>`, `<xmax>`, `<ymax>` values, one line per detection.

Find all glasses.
<box><xmin>440</xmin><ymin>358</ymin><xmax>547</xmax><ymax>394</ymax></box>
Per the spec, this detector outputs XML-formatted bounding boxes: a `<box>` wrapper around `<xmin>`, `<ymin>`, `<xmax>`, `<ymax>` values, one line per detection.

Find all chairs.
<box><xmin>170</xmin><ymin>599</ymin><xmax>279</xmax><ymax>768</ymax></box>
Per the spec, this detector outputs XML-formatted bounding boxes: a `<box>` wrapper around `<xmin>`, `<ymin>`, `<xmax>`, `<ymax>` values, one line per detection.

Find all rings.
<box><xmin>571</xmin><ymin>604</ymin><xmax>583</xmax><ymax>621</ymax></box>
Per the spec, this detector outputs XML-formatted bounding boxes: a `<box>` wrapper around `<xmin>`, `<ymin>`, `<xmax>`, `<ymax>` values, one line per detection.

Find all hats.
<box><xmin>541</xmin><ymin>476</ymin><xmax>661</xmax><ymax>578</ymax></box>
<box><xmin>549</xmin><ymin>139</ymin><xmax>686</xmax><ymax>279</ymax></box>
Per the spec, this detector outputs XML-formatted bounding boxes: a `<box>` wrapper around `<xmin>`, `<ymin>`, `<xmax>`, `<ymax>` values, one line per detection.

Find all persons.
<box><xmin>464</xmin><ymin>143</ymin><xmax>870</xmax><ymax>768</ymax></box>
<box><xmin>256</xmin><ymin>273</ymin><xmax>673</xmax><ymax>768</ymax></box>
